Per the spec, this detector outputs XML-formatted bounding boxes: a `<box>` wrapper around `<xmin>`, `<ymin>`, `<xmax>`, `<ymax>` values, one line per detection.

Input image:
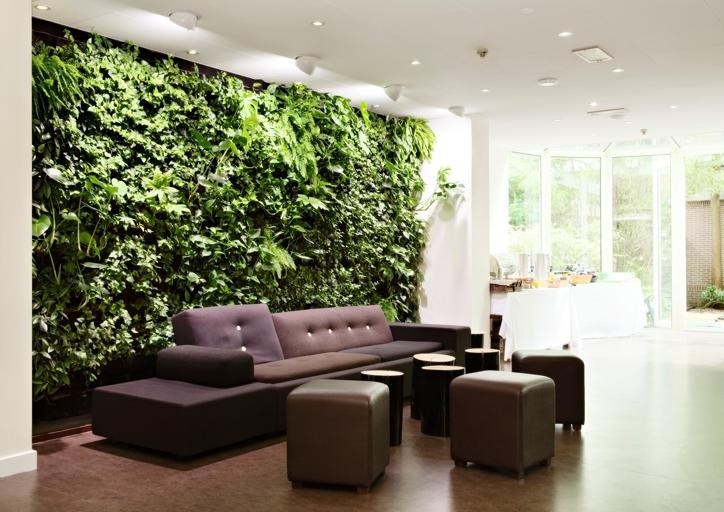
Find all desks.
<box><xmin>490</xmin><ymin>278</ymin><xmax>644</xmax><ymax>363</ymax></box>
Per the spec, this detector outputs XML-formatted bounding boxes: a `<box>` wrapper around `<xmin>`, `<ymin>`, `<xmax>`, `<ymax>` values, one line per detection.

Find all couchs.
<box><xmin>91</xmin><ymin>304</ymin><xmax>472</xmax><ymax>461</ymax></box>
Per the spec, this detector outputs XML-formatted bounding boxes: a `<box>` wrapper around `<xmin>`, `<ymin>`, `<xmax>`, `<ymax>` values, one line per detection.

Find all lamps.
<box><xmin>171</xmin><ymin>13</ymin><xmax>199</xmax><ymax>32</ymax></box>
<box><xmin>450</xmin><ymin>106</ymin><xmax>467</xmax><ymax>119</ymax></box>
<box><xmin>384</xmin><ymin>84</ymin><xmax>402</xmax><ymax>102</ymax></box>
<box><xmin>294</xmin><ymin>55</ymin><xmax>320</xmax><ymax>76</ymax></box>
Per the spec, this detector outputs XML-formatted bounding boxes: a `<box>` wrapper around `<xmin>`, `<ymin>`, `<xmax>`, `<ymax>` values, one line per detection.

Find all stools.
<box><xmin>449</xmin><ymin>370</ymin><xmax>557</xmax><ymax>479</ymax></box>
<box><xmin>285</xmin><ymin>378</ymin><xmax>391</xmax><ymax>494</ymax></box>
<box><xmin>360</xmin><ymin>370</ymin><xmax>404</xmax><ymax>447</ymax></box>
<box><xmin>512</xmin><ymin>349</ymin><xmax>585</xmax><ymax>432</ymax></box>
<box><xmin>410</xmin><ymin>348</ymin><xmax>500</xmax><ymax>438</ymax></box>
